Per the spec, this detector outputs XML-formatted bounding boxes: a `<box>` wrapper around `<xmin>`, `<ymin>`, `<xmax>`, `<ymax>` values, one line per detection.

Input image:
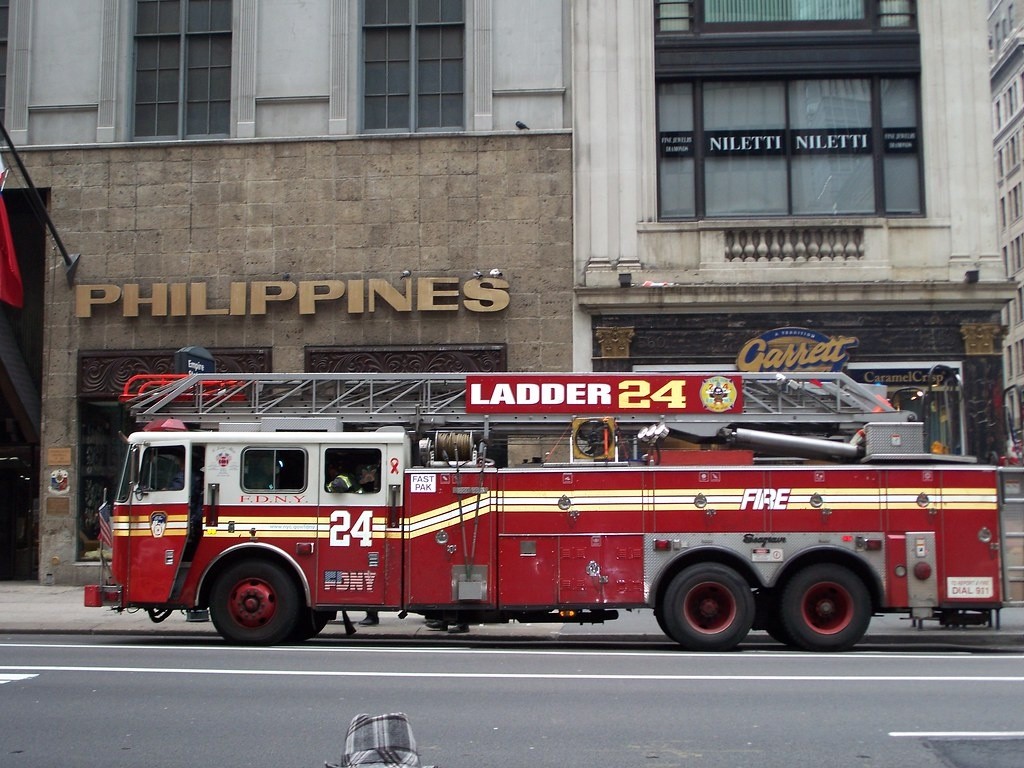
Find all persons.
<box><xmin>162</xmin><ymin>452</ymin><xmax>203</xmax><ymax>542</ymax></box>
<box><xmin>243</xmin><ymin>451</ymin><xmax>380</xmax><ymax>495</ymax></box>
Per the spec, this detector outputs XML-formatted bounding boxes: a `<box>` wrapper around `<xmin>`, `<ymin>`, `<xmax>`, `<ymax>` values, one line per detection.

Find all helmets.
<box><xmin>329</xmin><ymin>453</ymin><xmax>350</xmax><ymax>473</ymax></box>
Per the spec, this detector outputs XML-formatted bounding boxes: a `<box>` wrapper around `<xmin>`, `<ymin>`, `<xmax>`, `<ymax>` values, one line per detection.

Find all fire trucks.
<box><xmin>83</xmin><ymin>362</ymin><xmax>1014</xmax><ymax>653</ymax></box>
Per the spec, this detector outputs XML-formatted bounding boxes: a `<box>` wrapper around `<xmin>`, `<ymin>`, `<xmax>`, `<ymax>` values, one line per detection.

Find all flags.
<box><xmin>0</xmin><ymin>155</ymin><xmax>24</xmax><ymax>309</ymax></box>
<box><xmin>97</xmin><ymin>502</ymin><xmax>113</xmax><ymax>548</ymax></box>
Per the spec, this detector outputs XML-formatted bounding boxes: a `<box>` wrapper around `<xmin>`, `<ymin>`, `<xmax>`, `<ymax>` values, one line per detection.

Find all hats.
<box><xmin>324</xmin><ymin>711</ymin><xmax>437</xmax><ymax>768</ymax></box>
